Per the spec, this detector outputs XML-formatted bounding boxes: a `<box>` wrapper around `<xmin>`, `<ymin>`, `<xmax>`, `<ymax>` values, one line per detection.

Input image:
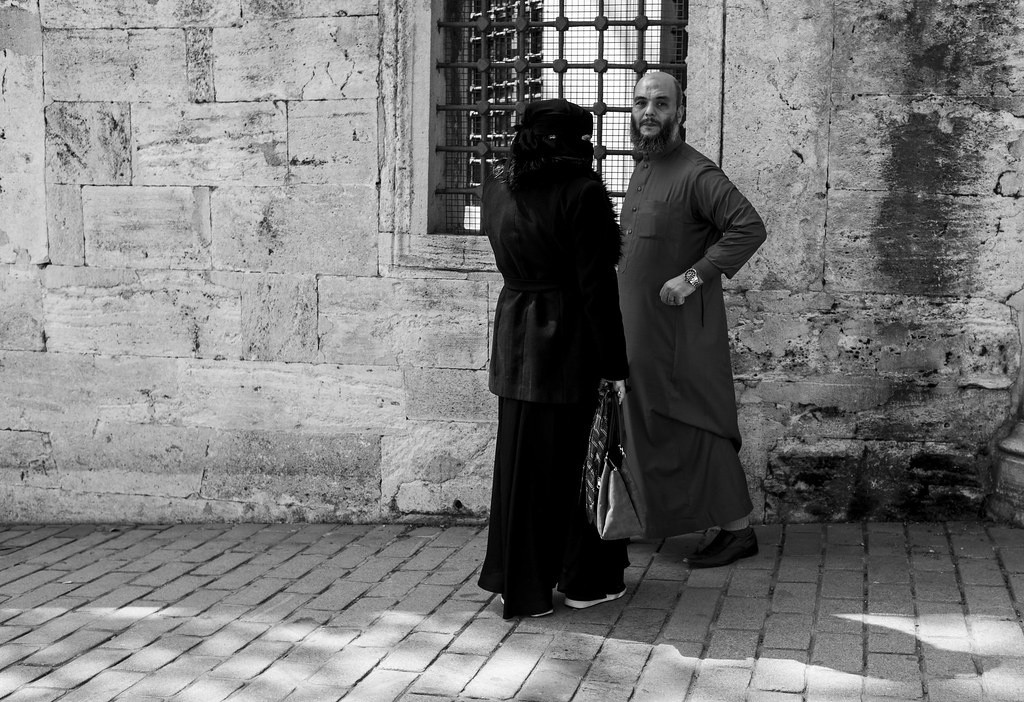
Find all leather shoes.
<box><xmin>682</xmin><ymin>527</ymin><xmax>759</xmax><ymax>568</ymax></box>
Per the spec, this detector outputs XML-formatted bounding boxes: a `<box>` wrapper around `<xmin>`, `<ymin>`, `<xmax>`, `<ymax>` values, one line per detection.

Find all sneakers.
<box><xmin>564</xmin><ymin>583</ymin><xmax>628</xmax><ymax>609</ymax></box>
<box><xmin>500</xmin><ymin>595</ymin><xmax>553</xmax><ymax>617</ymax></box>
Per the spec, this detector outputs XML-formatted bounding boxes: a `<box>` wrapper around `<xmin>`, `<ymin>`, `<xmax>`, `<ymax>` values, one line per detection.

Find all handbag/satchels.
<box><xmin>581</xmin><ymin>383</ymin><xmax>647</xmax><ymax>541</ymax></box>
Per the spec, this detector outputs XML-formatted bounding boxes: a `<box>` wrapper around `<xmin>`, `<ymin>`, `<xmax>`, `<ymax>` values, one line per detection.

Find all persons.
<box><xmin>478</xmin><ymin>100</ymin><xmax>629</xmax><ymax>621</ymax></box>
<box><xmin>616</xmin><ymin>71</ymin><xmax>768</xmax><ymax>596</ymax></box>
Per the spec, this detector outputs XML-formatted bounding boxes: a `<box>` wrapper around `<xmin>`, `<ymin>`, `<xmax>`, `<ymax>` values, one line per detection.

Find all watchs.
<box><xmin>684</xmin><ymin>269</ymin><xmax>700</xmax><ymax>289</ymax></box>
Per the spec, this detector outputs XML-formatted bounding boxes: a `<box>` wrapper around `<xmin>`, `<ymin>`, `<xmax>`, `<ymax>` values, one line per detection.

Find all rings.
<box><xmin>668</xmin><ymin>299</ymin><xmax>674</xmax><ymax>302</ymax></box>
